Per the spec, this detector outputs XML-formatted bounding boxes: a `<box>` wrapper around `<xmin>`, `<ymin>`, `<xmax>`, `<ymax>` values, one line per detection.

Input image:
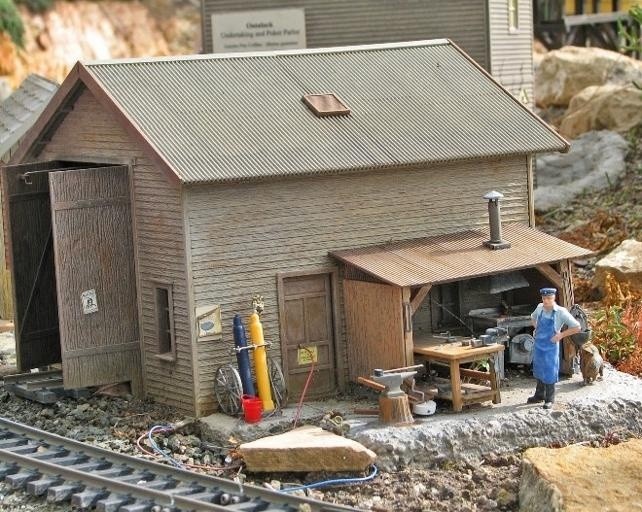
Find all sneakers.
<box><xmin>544</xmin><ymin>401</ymin><xmax>552</xmax><ymax>408</ymax></box>
<box><xmin>528</xmin><ymin>397</ymin><xmax>544</xmax><ymax>403</ymax></box>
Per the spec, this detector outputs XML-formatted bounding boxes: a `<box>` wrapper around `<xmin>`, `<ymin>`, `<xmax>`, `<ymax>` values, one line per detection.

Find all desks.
<box><xmin>413</xmin><ymin>332</ymin><xmax>505</xmax><ymax>413</ymax></box>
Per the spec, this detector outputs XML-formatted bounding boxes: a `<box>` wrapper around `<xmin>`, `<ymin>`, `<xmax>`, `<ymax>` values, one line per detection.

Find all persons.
<box><xmin>525</xmin><ymin>286</ymin><xmax>582</xmax><ymax>409</ymax></box>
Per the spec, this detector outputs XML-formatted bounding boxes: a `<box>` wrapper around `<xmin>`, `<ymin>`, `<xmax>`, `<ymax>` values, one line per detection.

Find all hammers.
<box><xmin>374</xmin><ymin>363</ymin><xmax>424</xmax><ymax>377</ymax></box>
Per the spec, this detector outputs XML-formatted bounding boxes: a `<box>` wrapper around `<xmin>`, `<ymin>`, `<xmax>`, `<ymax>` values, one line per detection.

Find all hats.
<box><xmin>540</xmin><ymin>288</ymin><xmax>557</xmax><ymax>296</ymax></box>
